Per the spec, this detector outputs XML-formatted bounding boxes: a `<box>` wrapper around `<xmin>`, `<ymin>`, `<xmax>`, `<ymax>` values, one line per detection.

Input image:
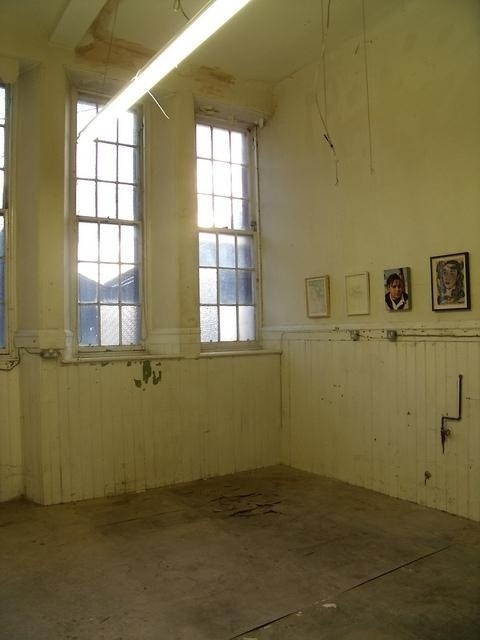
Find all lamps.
<box><xmin>75</xmin><ymin>0</ymin><xmax>253</xmax><ymax>152</ymax></box>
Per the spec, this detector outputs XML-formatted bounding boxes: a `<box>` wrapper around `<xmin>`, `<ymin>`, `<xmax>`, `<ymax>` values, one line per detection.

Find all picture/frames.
<box><xmin>304</xmin><ymin>252</ymin><xmax>472</xmax><ymax>318</ymax></box>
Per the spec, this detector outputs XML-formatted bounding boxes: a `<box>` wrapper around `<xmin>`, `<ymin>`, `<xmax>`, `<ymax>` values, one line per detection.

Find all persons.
<box><xmin>436</xmin><ymin>260</ymin><xmax>465</xmax><ymax>305</ymax></box>
<box><xmin>383</xmin><ymin>272</ymin><xmax>408</xmax><ymax>311</ymax></box>
